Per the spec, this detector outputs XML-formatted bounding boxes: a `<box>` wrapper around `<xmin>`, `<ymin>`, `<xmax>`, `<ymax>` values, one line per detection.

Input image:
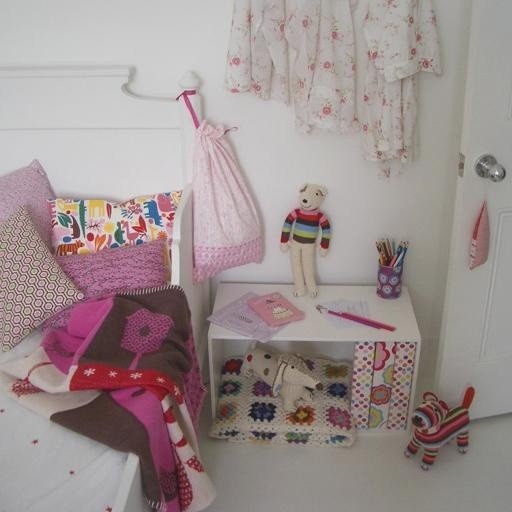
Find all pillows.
<box><xmin>42</xmin><ymin>239</ymin><xmax>166</xmax><ymax>341</ymax></box>
<box><xmin>0</xmin><ymin>205</ymin><xmax>84</xmax><ymax>355</ymax></box>
<box><xmin>0</xmin><ymin>159</ymin><xmax>56</xmax><ymax>243</ymax></box>
<box><xmin>46</xmin><ymin>190</ymin><xmax>183</xmax><ymax>257</ymax></box>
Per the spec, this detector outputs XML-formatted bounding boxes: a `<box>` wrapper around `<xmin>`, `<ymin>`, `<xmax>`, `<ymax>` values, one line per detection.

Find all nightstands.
<box><xmin>207</xmin><ymin>283</ymin><xmax>421</xmax><ymax>438</ymax></box>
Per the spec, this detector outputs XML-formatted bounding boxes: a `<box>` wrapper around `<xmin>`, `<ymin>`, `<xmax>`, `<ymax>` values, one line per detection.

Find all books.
<box><xmin>206</xmin><ymin>293</ymin><xmax>290</xmax><ymax>343</ymax></box>
<box><xmin>247</xmin><ymin>292</ymin><xmax>305</xmax><ymax>328</ymax></box>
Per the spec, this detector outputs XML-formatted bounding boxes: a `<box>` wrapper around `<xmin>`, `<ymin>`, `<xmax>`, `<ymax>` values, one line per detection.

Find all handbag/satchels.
<box><xmin>468</xmin><ymin>175</ymin><xmax>490</xmax><ymax>270</ymax></box>
<box><xmin>177</xmin><ymin>90</ymin><xmax>265</xmax><ymax>284</ymax></box>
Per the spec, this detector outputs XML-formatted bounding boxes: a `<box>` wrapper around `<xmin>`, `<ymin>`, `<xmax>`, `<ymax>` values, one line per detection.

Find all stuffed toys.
<box><xmin>240</xmin><ymin>340</ymin><xmax>323</xmax><ymax>412</ymax></box>
<box><xmin>403</xmin><ymin>387</ymin><xmax>475</xmax><ymax>471</ymax></box>
<box><xmin>280</xmin><ymin>184</ymin><xmax>330</xmax><ymax>298</ymax></box>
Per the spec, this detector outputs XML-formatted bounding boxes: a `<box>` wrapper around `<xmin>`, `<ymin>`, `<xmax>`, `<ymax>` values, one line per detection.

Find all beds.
<box><xmin>0</xmin><ymin>62</ymin><xmax>207</xmax><ymax>512</ymax></box>
